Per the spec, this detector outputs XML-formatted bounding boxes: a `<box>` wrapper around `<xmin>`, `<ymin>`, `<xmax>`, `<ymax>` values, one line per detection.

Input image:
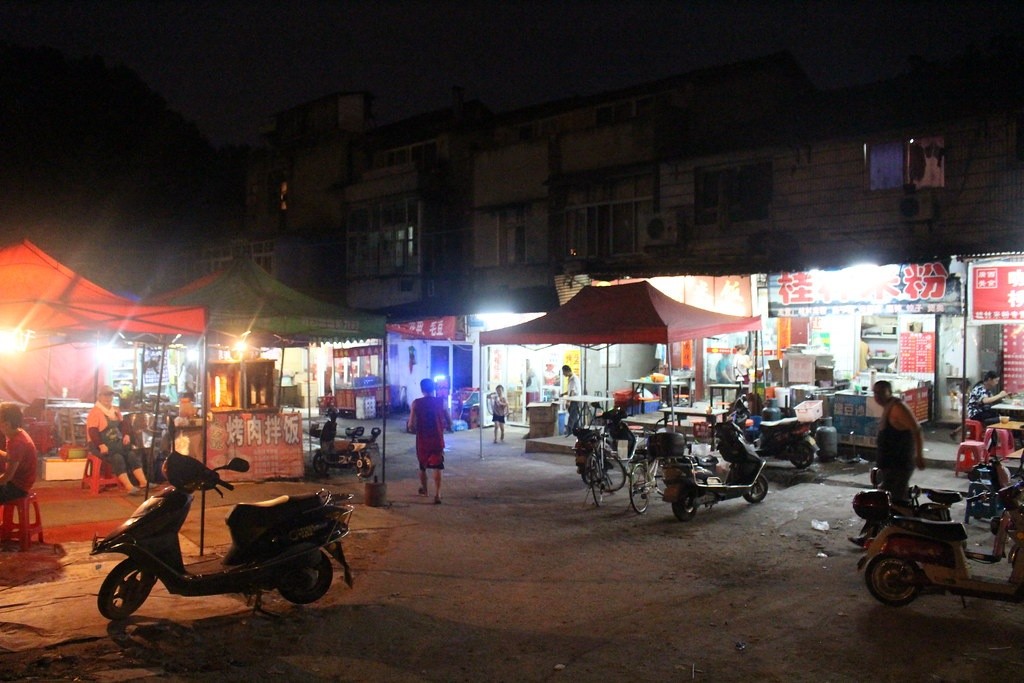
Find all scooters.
<box><xmin>313</xmin><ymin>405</ymin><xmax>381</xmax><ymax>482</ymax></box>
<box><xmin>727</xmin><ymin>393</ymin><xmax>820</xmax><ymax>469</ymax></box>
<box><xmin>847</xmin><ymin>427</ymin><xmax>1024</xmax><ymax>607</ymax></box>
<box><xmin>647</xmin><ymin>394</ymin><xmax>769</xmax><ymax>522</ymax></box>
<box><xmin>572</xmin><ymin>400</ymin><xmax>638</xmax><ymax>484</ymax></box>
<box><xmin>90</xmin><ymin>417</ymin><xmax>353</xmax><ymax>621</ymax></box>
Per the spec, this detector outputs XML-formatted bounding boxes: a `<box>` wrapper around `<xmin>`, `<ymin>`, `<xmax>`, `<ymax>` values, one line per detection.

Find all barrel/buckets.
<box><xmin>774</xmin><ymin>388</ymin><xmax>791</xmax><ymax>408</ymax></box>
<box><xmin>812</xmin><ymin>388</ymin><xmax>837</xmax><ymax>418</ymax></box>
<box><xmin>558</xmin><ymin>412</ymin><xmax>565</xmax><ymax>435</ymax></box>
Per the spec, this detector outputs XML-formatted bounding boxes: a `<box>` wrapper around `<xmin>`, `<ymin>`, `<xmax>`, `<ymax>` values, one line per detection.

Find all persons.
<box><xmin>872</xmin><ymin>380</ymin><xmax>926</xmax><ymax>509</ymax></box>
<box><xmin>177</xmin><ymin>364</ymin><xmax>194</xmax><ymax>401</ymax></box>
<box><xmin>487</xmin><ymin>385</ymin><xmax>509</xmax><ymax>443</ymax></box>
<box><xmin>408</xmin><ymin>378</ymin><xmax>453</xmax><ymax>505</ymax></box>
<box><xmin>87</xmin><ymin>385</ymin><xmax>158</xmax><ymax>496</ymax></box>
<box><xmin>559</xmin><ymin>365</ymin><xmax>582</xmax><ymax>436</ymax></box>
<box><xmin>860</xmin><ymin>340</ymin><xmax>870</xmax><ymax>373</ymax></box>
<box><xmin>967</xmin><ymin>370</ymin><xmax>1009</xmax><ymax>451</ymax></box>
<box><xmin>716</xmin><ymin>344</ymin><xmax>752</xmax><ymax>403</ymax></box>
<box><xmin>0</xmin><ymin>403</ymin><xmax>38</xmax><ymax>505</ymax></box>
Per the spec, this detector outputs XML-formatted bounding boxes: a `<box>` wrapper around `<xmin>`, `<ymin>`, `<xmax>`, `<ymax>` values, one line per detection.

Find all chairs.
<box><xmin>510</xmin><ymin>391</ymin><xmax>523</xmax><ymax>421</ymax></box>
<box><xmin>59</xmin><ymin>405</ymin><xmax>86</xmax><ymax>446</ymax></box>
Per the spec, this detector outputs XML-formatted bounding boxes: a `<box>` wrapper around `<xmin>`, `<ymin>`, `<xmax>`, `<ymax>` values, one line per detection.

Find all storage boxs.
<box><xmin>793</xmin><ymin>399</ymin><xmax>824</xmax><ymax>423</ymax></box>
<box><xmin>292</xmin><ymin>371</ymin><xmax>317</xmax><ymax>408</ymax></box>
<box><xmin>525</xmin><ymin>400</ymin><xmax>558</xmax><ymax>437</ymax></box>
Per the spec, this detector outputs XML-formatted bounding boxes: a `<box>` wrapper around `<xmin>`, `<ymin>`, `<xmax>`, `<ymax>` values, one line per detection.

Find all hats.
<box><xmin>100</xmin><ymin>385</ymin><xmax>115</xmax><ymax>396</ymax></box>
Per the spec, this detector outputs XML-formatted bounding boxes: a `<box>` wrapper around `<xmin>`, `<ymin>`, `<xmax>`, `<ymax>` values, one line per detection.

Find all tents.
<box><xmin>43</xmin><ymin>251</ymin><xmax>389</xmax><ymax>497</ymax></box>
<box><xmin>0</xmin><ymin>235</ymin><xmax>211</xmax><ymax>558</ymax></box>
<box><xmin>477</xmin><ymin>280</ymin><xmax>763</xmax><ymax>463</ymax></box>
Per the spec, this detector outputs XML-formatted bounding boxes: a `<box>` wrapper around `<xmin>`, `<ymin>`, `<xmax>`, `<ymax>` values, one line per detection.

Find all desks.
<box><xmin>1007</xmin><ymin>447</ymin><xmax>1024</xmax><ymax>460</ymax></box>
<box><xmin>987</xmin><ymin>421</ymin><xmax>1024</xmax><ymax>446</ymax></box>
<box><xmin>991</xmin><ymin>398</ymin><xmax>1024</xmax><ymax>419</ymax></box>
<box><xmin>563</xmin><ymin>396</ymin><xmax>612</xmax><ymax>433</ymax></box>
<box><xmin>625</xmin><ymin>379</ymin><xmax>686</xmax><ymax>415</ymax></box>
<box><xmin>660</xmin><ymin>405</ymin><xmax>728</xmax><ymax>451</ymax></box>
<box><xmin>707</xmin><ymin>383</ymin><xmax>747</xmax><ymax>412</ymax></box>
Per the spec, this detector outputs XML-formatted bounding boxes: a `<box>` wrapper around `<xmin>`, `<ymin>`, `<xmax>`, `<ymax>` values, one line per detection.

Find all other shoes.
<box><xmin>418</xmin><ymin>487</ymin><xmax>429</xmax><ymax>497</ymax></box>
<box><xmin>848</xmin><ymin>534</ymin><xmax>868</xmax><ymax>547</ymax></box>
<box><xmin>434</xmin><ymin>496</ymin><xmax>442</xmax><ymax>504</ymax></box>
<box><xmin>124</xmin><ymin>483</ymin><xmax>140</xmax><ymax>494</ymax></box>
<box><xmin>951</xmin><ymin>433</ymin><xmax>957</xmax><ymax>441</ymax></box>
<box><xmin>138</xmin><ymin>481</ymin><xmax>157</xmax><ymax>489</ymax></box>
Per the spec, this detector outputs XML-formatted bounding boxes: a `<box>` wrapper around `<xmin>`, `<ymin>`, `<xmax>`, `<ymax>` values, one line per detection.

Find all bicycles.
<box><xmin>629</xmin><ymin>414</ymin><xmax>668</xmax><ymax>514</ymax></box>
<box><xmin>581</xmin><ymin>425</ymin><xmax>626</xmax><ymax>508</ymax></box>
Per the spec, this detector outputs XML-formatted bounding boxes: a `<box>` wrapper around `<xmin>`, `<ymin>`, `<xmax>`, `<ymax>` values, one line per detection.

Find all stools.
<box><xmin>955</xmin><ymin>419</ymin><xmax>1014</xmax><ymax>524</ymax></box>
<box><xmin>0</xmin><ymin>491</ymin><xmax>44</xmax><ymax>552</ymax></box>
<box><xmin>20</xmin><ymin>416</ymin><xmax>54</xmax><ymax>453</ymax></box>
<box><xmin>622</xmin><ymin>394</ymin><xmax>734</xmax><ymax>441</ymax></box>
<box><xmin>81</xmin><ymin>454</ymin><xmax>126</xmax><ymax>493</ymax></box>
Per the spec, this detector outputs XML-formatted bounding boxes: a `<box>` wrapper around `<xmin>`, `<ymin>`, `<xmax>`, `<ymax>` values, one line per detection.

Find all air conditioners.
<box><xmin>636</xmin><ymin>207</ymin><xmax>687</xmax><ymax>251</ymax></box>
<box><xmin>898</xmin><ymin>190</ymin><xmax>939</xmax><ymax>224</ymax></box>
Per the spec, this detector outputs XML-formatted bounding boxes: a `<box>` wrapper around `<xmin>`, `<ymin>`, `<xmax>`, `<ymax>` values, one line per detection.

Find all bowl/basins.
<box><xmin>65</xmin><ymin>448</ymin><xmax>88</xmax><ymax>458</ymax></box>
<box><xmin>1001</xmin><ymin>399</ymin><xmax>1014</xmax><ymax>403</ymax></box>
<box><xmin>1000</xmin><ymin>416</ymin><xmax>1009</xmax><ymax>424</ymax></box>
